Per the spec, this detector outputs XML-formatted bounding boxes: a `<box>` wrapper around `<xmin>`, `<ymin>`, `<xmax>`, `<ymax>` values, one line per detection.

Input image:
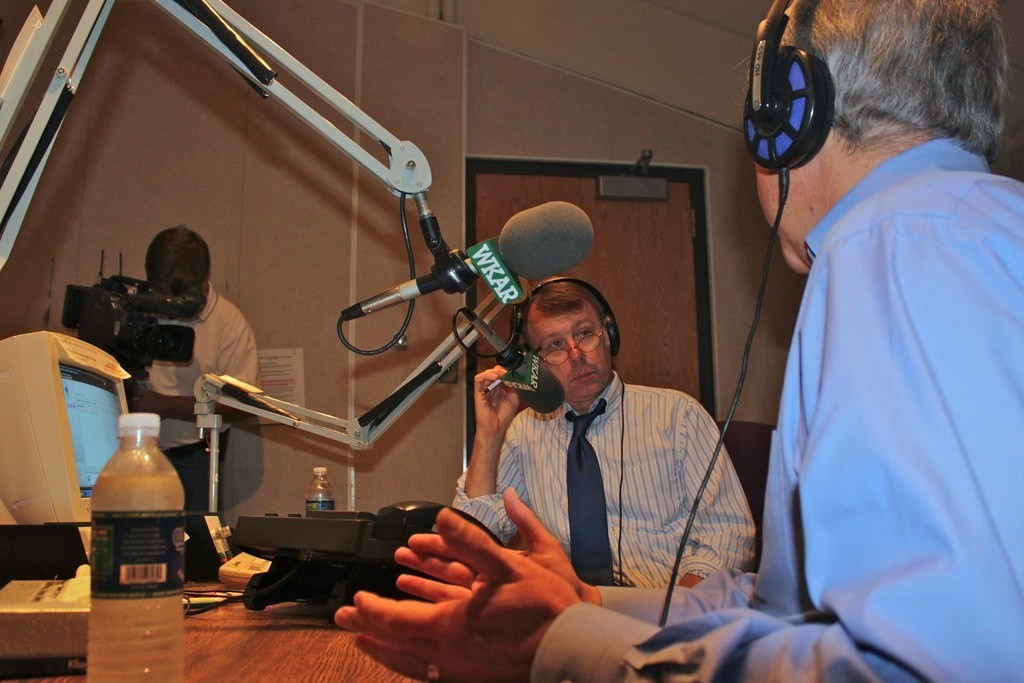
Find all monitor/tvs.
<box><xmin>0</xmin><ymin>329</ymin><xmax>128</xmax><ymax>562</ymax></box>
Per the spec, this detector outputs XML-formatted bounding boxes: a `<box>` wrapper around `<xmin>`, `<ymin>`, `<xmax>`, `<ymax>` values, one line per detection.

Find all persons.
<box><xmin>126</xmin><ymin>222</ymin><xmax>261</xmax><ymax>583</ymax></box>
<box><xmin>332</xmin><ymin>0</ymin><xmax>1024</xmax><ymax>683</ymax></box>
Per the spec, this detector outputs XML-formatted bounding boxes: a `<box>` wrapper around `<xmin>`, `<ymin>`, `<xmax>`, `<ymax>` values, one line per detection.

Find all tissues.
<box><xmin>0</xmin><ymin>564</ymin><xmax>91</xmax><ymax>658</ymax></box>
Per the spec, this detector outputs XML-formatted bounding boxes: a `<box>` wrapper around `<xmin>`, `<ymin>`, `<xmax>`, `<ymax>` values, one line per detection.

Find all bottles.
<box><xmin>306</xmin><ymin>467</ymin><xmax>334</xmax><ymax>511</ymax></box>
<box><xmin>87</xmin><ymin>413</ymin><xmax>188</xmax><ymax>683</ymax></box>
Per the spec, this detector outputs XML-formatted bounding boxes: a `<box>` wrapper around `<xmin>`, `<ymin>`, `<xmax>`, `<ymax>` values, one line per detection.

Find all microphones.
<box><xmin>459</xmin><ymin>305</ymin><xmax>565</xmax><ymax>415</ymax></box>
<box><xmin>338</xmin><ymin>200</ymin><xmax>596</xmax><ymax>324</ymax></box>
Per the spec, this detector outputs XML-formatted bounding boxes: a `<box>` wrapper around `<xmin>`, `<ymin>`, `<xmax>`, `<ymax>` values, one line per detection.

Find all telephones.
<box><xmin>232</xmin><ymin>499</ymin><xmax>507</xmax><ymax>624</ymax></box>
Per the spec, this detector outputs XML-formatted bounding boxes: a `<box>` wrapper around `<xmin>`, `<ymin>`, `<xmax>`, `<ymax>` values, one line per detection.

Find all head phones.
<box><xmin>510</xmin><ymin>276</ymin><xmax>620</xmax><ymax>357</ymax></box>
<box><xmin>741</xmin><ymin>0</ymin><xmax>837</xmax><ymax>175</ymax></box>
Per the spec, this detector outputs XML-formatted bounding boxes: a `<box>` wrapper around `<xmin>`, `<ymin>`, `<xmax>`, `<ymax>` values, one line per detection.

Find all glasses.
<box><xmin>535</xmin><ymin>322</ymin><xmax>604</xmax><ymax>366</ymax></box>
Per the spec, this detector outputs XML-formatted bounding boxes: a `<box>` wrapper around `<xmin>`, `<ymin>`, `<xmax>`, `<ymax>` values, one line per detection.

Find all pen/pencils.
<box><xmin>482</xmin><ymin>379</ymin><xmax>501</xmax><ymax>394</ymax></box>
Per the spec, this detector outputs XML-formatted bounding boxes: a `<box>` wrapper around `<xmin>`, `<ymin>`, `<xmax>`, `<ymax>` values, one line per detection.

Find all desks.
<box><xmin>0</xmin><ymin>581</ymin><xmax>441</xmax><ymax>683</ymax></box>
